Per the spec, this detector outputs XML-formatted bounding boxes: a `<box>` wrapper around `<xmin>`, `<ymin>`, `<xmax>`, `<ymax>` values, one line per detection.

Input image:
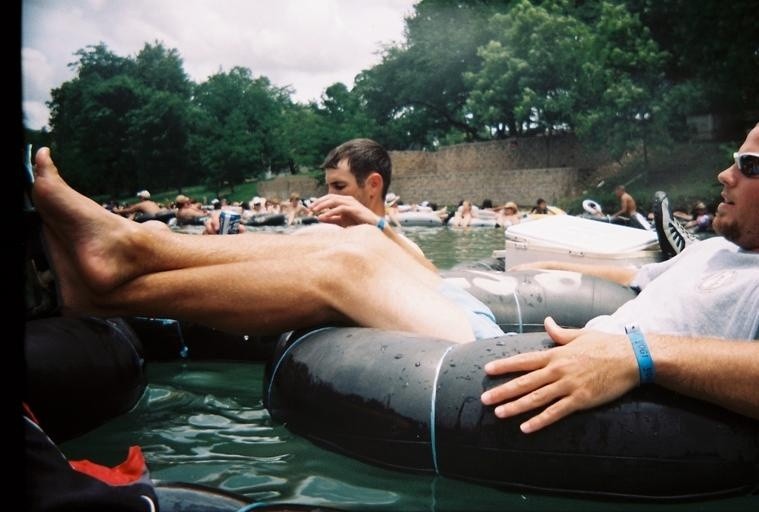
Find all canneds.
<box><xmin>218</xmin><ymin>211</ymin><xmax>241</xmax><ymax>235</ymax></box>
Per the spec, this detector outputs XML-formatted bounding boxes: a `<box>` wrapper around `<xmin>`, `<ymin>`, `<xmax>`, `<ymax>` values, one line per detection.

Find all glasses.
<box><xmin>732</xmin><ymin>152</ymin><xmax>758</xmax><ymax>177</ymax></box>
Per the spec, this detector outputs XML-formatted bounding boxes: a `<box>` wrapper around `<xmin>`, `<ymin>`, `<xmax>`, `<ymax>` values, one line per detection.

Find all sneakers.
<box><xmin>653</xmin><ymin>190</ymin><xmax>703</xmax><ymax>259</ymax></box>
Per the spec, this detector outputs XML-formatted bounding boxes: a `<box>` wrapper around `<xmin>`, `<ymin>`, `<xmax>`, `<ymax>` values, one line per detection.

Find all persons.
<box><xmin>141</xmin><ymin>138</ymin><xmax>441</xmax><ymax>274</ymax></box>
<box><xmin>31</xmin><ymin>118</ymin><xmax>759</xmax><ymax>438</ymax></box>
<box><xmin>87</xmin><ymin>186</ymin><xmax>328</xmax><ymax>228</ymax></box>
<box><xmin>387</xmin><ymin>185</ymin><xmax>713</xmax><ymax>227</ymax></box>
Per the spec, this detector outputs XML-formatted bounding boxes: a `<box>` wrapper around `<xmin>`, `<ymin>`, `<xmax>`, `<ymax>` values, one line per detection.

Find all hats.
<box><xmin>503</xmin><ymin>202</ymin><xmax>516</xmax><ymax>210</ymax></box>
<box><xmin>249</xmin><ymin>196</ymin><xmax>266</xmax><ymax>208</ymax></box>
<box><xmin>386</xmin><ymin>193</ymin><xmax>399</xmax><ymax>206</ymax></box>
<box><xmin>176</xmin><ymin>195</ymin><xmax>189</xmax><ymax>203</ymax></box>
<box><xmin>137</xmin><ymin>191</ymin><xmax>150</xmax><ymax>198</ymax></box>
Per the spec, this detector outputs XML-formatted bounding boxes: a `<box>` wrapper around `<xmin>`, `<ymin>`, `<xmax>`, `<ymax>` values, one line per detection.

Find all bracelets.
<box><xmin>624</xmin><ymin>323</ymin><xmax>657</xmax><ymax>386</ymax></box>
<box><xmin>377</xmin><ymin>218</ymin><xmax>385</xmax><ymax>232</ymax></box>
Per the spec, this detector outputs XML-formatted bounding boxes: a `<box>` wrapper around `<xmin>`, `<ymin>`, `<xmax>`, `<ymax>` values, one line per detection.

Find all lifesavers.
<box><xmin>261</xmin><ymin>269</ymin><xmax>759</xmax><ymax>506</ymax></box>
<box><xmin>120</xmin><ymin>301</ymin><xmax>272</xmax><ymax>363</ymax></box>
<box><xmin>25</xmin><ymin>303</ymin><xmax>151</xmax><ymax>443</ymax></box>
<box><xmin>526</xmin><ymin>203</ymin><xmax>567</xmax><ymax>222</ymax></box>
<box><xmin>582</xmin><ymin>200</ymin><xmax>603</xmax><ymax>215</ymax></box>
<box><xmin>132</xmin><ymin>205</ymin><xmax>321</xmax><ymax>226</ymax></box>
<box><xmin>102</xmin><ymin>484</ymin><xmax>265</xmax><ymax>512</ymax></box>
<box><xmin>393</xmin><ymin>205</ymin><xmax>504</xmax><ymax>228</ymax></box>
